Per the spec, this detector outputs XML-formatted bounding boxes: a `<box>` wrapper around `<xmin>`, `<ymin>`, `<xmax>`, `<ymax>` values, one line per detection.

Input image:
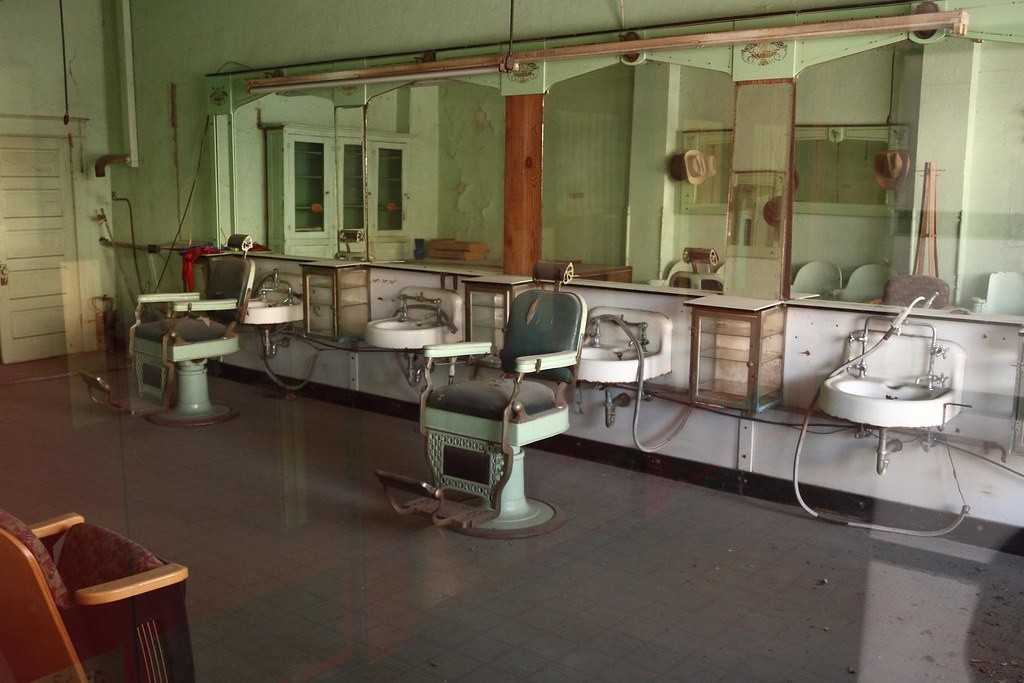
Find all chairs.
<box><xmin>0</xmin><ymin>510</ymin><xmax>195</xmax><ymax>683</ymax></box>
<box><xmin>973</xmin><ymin>271</ymin><xmax>1024</xmax><ymax>316</ymax></box>
<box><xmin>668</xmin><ymin>247</ymin><xmax>724</xmax><ymax>292</ymax></box>
<box><xmin>838</xmin><ymin>265</ymin><xmax>898</xmax><ymax>305</ymax></box>
<box><xmin>790</xmin><ymin>261</ymin><xmax>842</xmax><ymax>300</ymax></box>
<box><xmin>77</xmin><ymin>255</ymin><xmax>256</xmax><ymax>427</ymax></box>
<box><xmin>371</xmin><ymin>289</ymin><xmax>587</xmax><ymax>540</ymax></box>
<box><xmin>869</xmin><ymin>275</ymin><xmax>954</xmax><ymax>311</ymax></box>
<box><xmin>335</xmin><ymin>230</ymin><xmax>375</xmax><ymax>260</ymax></box>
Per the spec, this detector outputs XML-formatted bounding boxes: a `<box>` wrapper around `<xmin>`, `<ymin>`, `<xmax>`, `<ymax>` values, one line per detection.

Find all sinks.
<box><xmin>242</xmin><ymin>299</ymin><xmax>303</xmax><ymax>326</ymax></box>
<box><xmin>365</xmin><ymin>318</ymin><xmax>462</xmax><ymax>351</ymax></box>
<box><xmin>574</xmin><ymin>344</ymin><xmax>671</xmax><ymax>382</ymax></box>
<box><xmin>818</xmin><ymin>377</ymin><xmax>961</xmax><ymax>427</ymax></box>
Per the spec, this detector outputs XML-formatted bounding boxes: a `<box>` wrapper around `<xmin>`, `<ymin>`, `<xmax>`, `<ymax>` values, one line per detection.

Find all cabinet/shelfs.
<box><xmin>683</xmin><ymin>295</ymin><xmax>787</xmax><ymax>414</ymax></box>
<box><xmin>461</xmin><ymin>277</ymin><xmax>533</xmax><ymax>370</ymax></box>
<box><xmin>300</xmin><ymin>262</ymin><xmax>371</xmax><ymax>342</ymax></box>
<box><xmin>258</xmin><ymin>120</ymin><xmax>421</xmax><ymax>259</ymax></box>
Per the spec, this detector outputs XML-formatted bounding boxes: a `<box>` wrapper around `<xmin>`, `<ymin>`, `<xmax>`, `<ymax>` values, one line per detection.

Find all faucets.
<box><xmin>424</xmin><ymin>298</ymin><xmax>446</xmax><ymax>328</ymax></box>
<box><xmin>258</xmin><ymin>283</ymin><xmax>269</xmax><ymax>300</ymax></box>
<box><xmin>588</xmin><ymin>318</ymin><xmax>602</xmax><ymax>348</ymax></box>
<box><xmin>397</xmin><ymin>294</ymin><xmax>410</xmax><ymax>322</ymax></box>
<box><xmin>845</xmin><ymin>359</ymin><xmax>868</xmax><ymax>379</ymax></box>
<box><xmin>628</xmin><ymin>322</ymin><xmax>649</xmax><ymax>352</ymax></box>
<box><xmin>283</xmin><ymin>286</ymin><xmax>296</xmax><ymax>305</ymax></box>
<box><xmin>915</xmin><ymin>372</ymin><xmax>948</xmax><ymax>390</ymax></box>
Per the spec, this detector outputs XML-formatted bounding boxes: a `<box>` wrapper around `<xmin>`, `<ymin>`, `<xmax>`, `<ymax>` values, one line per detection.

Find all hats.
<box><xmin>684</xmin><ymin>149</ymin><xmax>709</xmax><ymax>185</ymax></box>
<box><xmin>874</xmin><ymin>150</ymin><xmax>911</xmax><ymax>190</ymax></box>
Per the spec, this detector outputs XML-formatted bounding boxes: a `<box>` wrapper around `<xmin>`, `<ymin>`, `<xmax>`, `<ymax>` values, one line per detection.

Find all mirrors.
<box><xmin>792</xmin><ymin>39</ymin><xmax>1024</xmax><ymax>315</ymax></box>
<box><xmin>541</xmin><ymin>60</ymin><xmax>737</xmax><ymax>294</ymax></box>
<box><xmin>233</xmin><ymin>79</ymin><xmax>505</xmax><ymax>270</ymax></box>
<box><xmin>680</xmin><ymin>125</ymin><xmax>898</xmax><ymax>218</ymax></box>
<box><xmin>722</xmin><ymin>79</ymin><xmax>794</xmax><ymax>301</ymax></box>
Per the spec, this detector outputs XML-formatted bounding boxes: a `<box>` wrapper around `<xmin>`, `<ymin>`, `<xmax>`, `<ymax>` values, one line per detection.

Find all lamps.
<box><xmin>246</xmin><ymin>57</ymin><xmax>519</xmax><ymax>93</ymax></box>
